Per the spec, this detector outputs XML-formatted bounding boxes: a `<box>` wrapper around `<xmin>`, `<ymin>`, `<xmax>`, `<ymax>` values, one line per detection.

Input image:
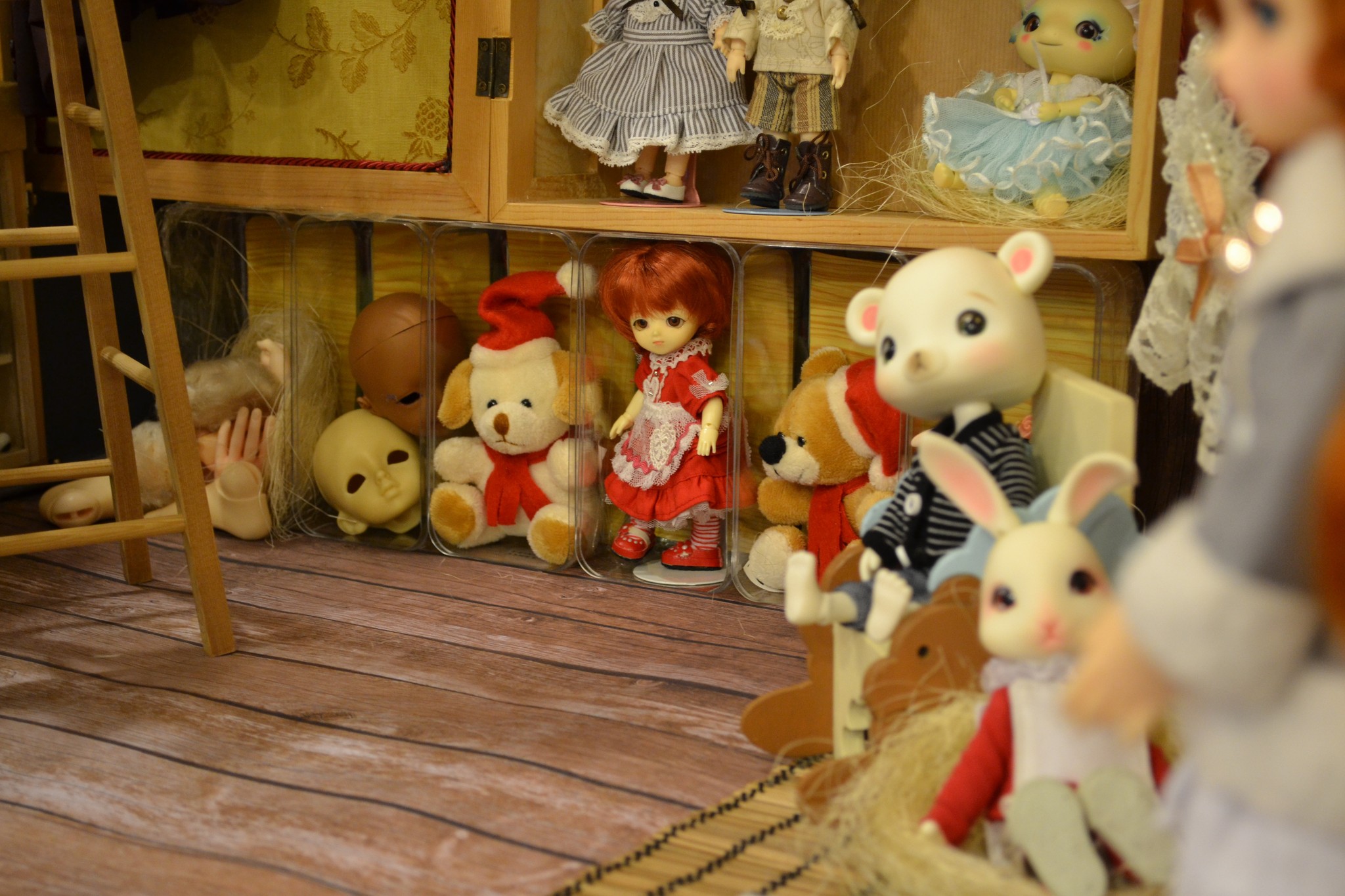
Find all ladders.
<box><xmin>1</xmin><ymin>0</ymin><xmax>242</xmax><ymax>657</ymax></box>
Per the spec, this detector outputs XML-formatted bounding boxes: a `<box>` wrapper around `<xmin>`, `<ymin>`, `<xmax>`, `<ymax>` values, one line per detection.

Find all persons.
<box><xmin>1054</xmin><ymin>0</ymin><xmax>1345</xmax><ymax>896</ymax></box>
<box><xmin>723</xmin><ymin>0</ymin><xmax>868</xmax><ymax>214</ymax></box>
<box><xmin>542</xmin><ymin>0</ymin><xmax>763</xmax><ymax>204</ymax></box>
<box><xmin>314</xmin><ymin>408</ymin><xmax>427</xmax><ymax>536</ymax></box>
<box><xmin>349</xmin><ymin>291</ymin><xmax>472</xmax><ymax>439</ymax></box>
<box><xmin>597</xmin><ymin>243</ymin><xmax>765</xmax><ymax>572</ymax></box>
<box><xmin>38</xmin><ymin>336</ymin><xmax>289</xmax><ymax>541</ymax></box>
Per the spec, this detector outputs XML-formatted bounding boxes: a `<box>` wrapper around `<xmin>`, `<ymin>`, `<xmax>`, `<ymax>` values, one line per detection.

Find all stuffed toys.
<box><xmin>426</xmin><ymin>259</ymin><xmax>620</xmax><ymax>567</ymax></box>
<box><xmin>748</xmin><ymin>345</ymin><xmax>923</xmax><ymax>591</ymax></box>
<box><xmin>913</xmin><ymin>428</ymin><xmax>1171</xmax><ymax>896</ymax></box>
<box><xmin>784</xmin><ymin>229</ymin><xmax>1056</xmax><ymax>641</ymax></box>
<box><xmin>916</xmin><ymin>0</ymin><xmax>1141</xmax><ymax>219</ymax></box>
